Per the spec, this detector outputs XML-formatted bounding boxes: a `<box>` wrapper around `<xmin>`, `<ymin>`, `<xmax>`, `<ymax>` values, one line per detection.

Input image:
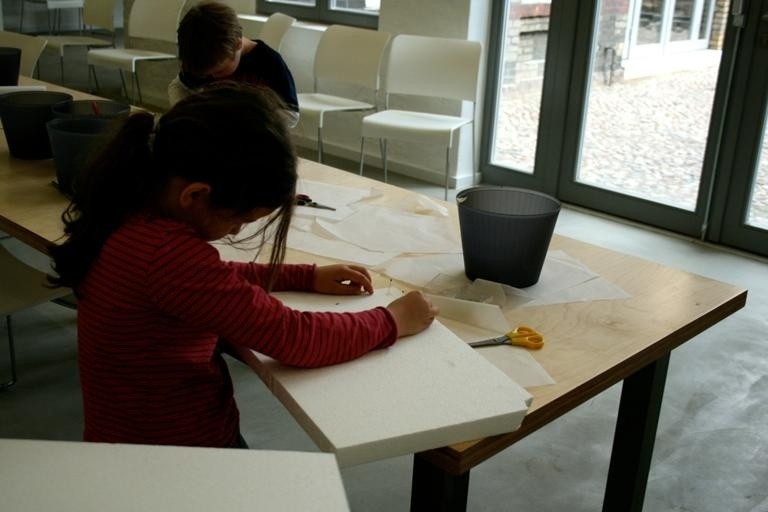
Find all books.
<box><xmin>233</xmin><ymin>279</ymin><xmax>533</xmax><ymax>464</ymax></box>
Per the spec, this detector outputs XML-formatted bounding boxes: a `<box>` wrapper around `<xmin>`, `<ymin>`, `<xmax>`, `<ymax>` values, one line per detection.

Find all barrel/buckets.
<box><xmin>456</xmin><ymin>186</ymin><xmax>563</xmax><ymax>289</ymax></box>
<box><xmin>0</xmin><ymin>46</ymin><xmax>132</xmax><ymax>196</ymax></box>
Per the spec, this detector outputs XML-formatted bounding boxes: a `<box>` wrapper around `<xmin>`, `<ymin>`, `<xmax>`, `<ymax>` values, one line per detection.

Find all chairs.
<box><xmin>86</xmin><ymin>1</ymin><xmax>187</xmax><ymax>108</ymax></box>
<box><xmin>293</xmin><ymin>23</ymin><xmax>394</xmax><ymax>170</ymax></box>
<box><xmin>35</xmin><ymin>1</ymin><xmax>117</xmax><ymax>94</ymax></box>
<box><xmin>256</xmin><ymin>11</ymin><xmax>296</xmax><ymax>52</ymax></box>
<box><xmin>356</xmin><ymin>33</ymin><xmax>483</xmax><ymax>202</ymax></box>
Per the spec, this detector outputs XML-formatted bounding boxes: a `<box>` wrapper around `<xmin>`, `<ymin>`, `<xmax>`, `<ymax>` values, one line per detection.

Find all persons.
<box><xmin>48</xmin><ymin>82</ymin><xmax>440</xmax><ymax>447</ymax></box>
<box><xmin>168</xmin><ymin>1</ymin><xmax>300</xmax><ymax>128</ymax></box>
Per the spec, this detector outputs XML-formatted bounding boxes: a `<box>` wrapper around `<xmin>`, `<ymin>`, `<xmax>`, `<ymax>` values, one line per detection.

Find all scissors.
<box><xmin>466</xmin><ymin>324</ymin><xmax>545</xmax><ymax>349</ymax></box>
<box><xmin>293</xmin><ymin>193</ymin><xmax>336</xmax><ymax>211</ymax></box>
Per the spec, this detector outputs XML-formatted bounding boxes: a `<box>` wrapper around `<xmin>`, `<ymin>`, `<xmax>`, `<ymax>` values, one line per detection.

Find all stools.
<box><xmin>0</xmin><ymin>241</ymin><xmax>78</xmax><ymax>390</ymax></box>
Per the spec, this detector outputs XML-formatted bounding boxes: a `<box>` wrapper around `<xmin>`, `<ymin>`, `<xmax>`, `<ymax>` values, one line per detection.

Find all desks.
<box><xmin>16</xmin><ymin>0</ymin><xmax>85</xmax><ymax>37</ymax></box>
<box><xmin>0</xmin><ymin>72</ymin><xmax>751</xmax><ymax>512</ymax></box>
